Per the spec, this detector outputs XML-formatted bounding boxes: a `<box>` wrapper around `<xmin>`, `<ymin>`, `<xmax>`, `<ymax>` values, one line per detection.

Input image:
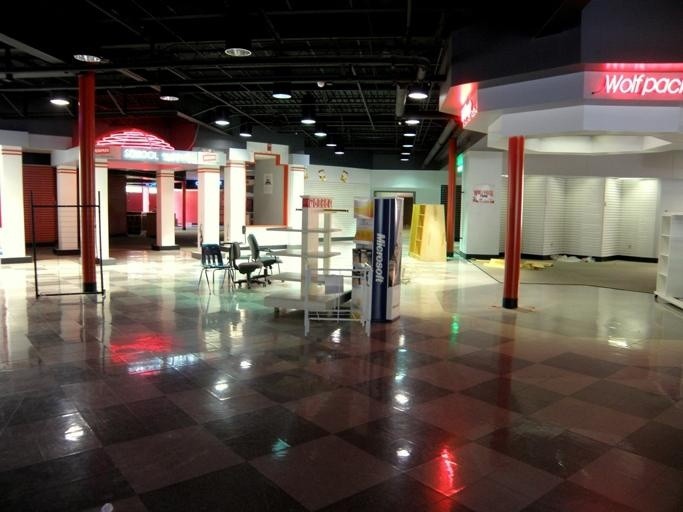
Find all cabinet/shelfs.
<box><xmin>407</xmin><ymin>203</ymin><xmax>447</xmax><ymax>263</ymax></box>
<box><xmin>265</xmin><ymin>194</ymin><xmax>354</xmax><ymax>323</ymax></box>
<box><xmin>651</xmin><ymin>209</ymin><xmax>683</xmax><ymax>309</ymax></box>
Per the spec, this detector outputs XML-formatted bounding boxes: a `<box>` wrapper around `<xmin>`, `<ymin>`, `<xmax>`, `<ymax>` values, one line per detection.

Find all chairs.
<box><xmin>196</xmin><ymin>233</ymin><xmax>284</xmax><ymax>294</ymax></box>
